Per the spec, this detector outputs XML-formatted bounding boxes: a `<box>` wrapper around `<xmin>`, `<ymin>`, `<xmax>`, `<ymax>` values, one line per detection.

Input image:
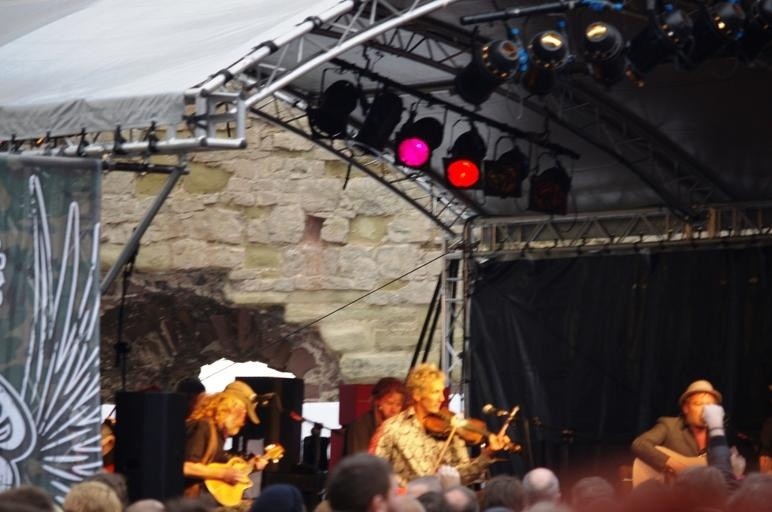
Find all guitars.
<box><xmin>205</xmin><ymin>442</ymin><xmax>285</xmax><ymax>507</ymax></box>
<box><xmin>617</xmin><ymin>446</ymin><xmax>744</xmax><ymax>495</ymax></box>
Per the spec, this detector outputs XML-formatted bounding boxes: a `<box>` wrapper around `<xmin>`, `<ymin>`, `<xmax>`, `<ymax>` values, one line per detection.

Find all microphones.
<box><xmin>482</xmin><ymin>403</ymin><xmax>510</xmax><ymax>417</ymax></box>
<box><xmin>723</xmin><ymin>414</ymin><xmax>753</xmax><ymax>442</ymax></box>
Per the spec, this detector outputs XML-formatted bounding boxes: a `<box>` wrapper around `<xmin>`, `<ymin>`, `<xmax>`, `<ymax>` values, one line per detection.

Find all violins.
<box><xmin>424</xmin><ymin>408</ymin><xmax>519</xmax><ymax>453</ymax></box>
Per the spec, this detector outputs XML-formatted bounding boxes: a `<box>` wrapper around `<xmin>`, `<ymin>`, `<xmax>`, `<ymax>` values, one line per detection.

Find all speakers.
<box><xmin>114</xmin><ymin>389</ymin><xmax>203</xmax><ymax>505</ymax></box>
<box><xmin>232</xmin><ymin>377</ymin><xmax>304</xmax><ymax>470</ymax></box>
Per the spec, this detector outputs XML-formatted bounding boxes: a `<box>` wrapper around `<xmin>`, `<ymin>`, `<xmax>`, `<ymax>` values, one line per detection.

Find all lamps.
<box><xmin>306</xmin><ymin>80</ymin><xmax>573</xmax><ymax>216</ymax></box>
<box><xmin>453</xmin><ymin>0</ymin><xmax>772</xmax><ymax>105</ymax></box>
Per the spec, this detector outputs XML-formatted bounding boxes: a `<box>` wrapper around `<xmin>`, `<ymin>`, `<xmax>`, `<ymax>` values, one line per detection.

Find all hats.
<box><xmin>226</xmin><ymin>382</ymin><xmax>260</xmax><ymax>425</ymax></box>
<box><xmin>681</xmin><ymin>380</ymin><xmax>721</xmax><ymax>405</ymax></box>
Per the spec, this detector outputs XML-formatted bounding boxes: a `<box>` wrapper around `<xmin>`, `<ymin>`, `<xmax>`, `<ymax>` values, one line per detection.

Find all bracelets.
<box><xmin>707</xmin><ymin>425</ymin><xmax>724</xmax><ymax>432</ymax></box>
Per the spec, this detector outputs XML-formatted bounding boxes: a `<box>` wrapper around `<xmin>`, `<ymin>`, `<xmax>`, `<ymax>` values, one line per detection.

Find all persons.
<box><xmin>626</xmin><ymin>378</ymin><xmax>746</xmax><ymax>483</ymax></box>
<box><xmin>0</xmin><ymin>450</ymin><xmax>771</xmax><ymax>512</ymax></box>
<box><xmin>365</xmin><ymin>361</ymin><xmax>521</xmax><ymax>481</ymax></box>
<box><xmin>161</xmin><ymin>386</ymin><xmax>271</xmax><ymax>508</ymax></box>
<box><xmin>339</xmin><ymin>376</ymin><xmax>407</xmax><ymax>457</ymax></box>
<box><xmin>698</xmin><ymin>403</ymin><xmax>740</xmax><ymax>491</ymax></box>
